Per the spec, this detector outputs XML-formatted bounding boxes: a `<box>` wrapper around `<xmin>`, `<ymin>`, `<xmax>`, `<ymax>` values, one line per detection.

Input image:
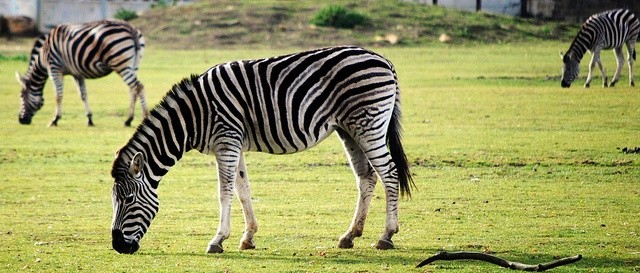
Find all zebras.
<box><xmin>15</xmin><ymin>19</ymin><xmax>149</xmax><ymax>126</ymax></box>
<box><xmin>110</xmin><ymin>44</ymin><xmax>420</xmax><ymax>254</ymax></box>
<box><xmin>560</xmin><ymin>9</ymin><xmax>640</xmax><ymax>88</ymax></box>
